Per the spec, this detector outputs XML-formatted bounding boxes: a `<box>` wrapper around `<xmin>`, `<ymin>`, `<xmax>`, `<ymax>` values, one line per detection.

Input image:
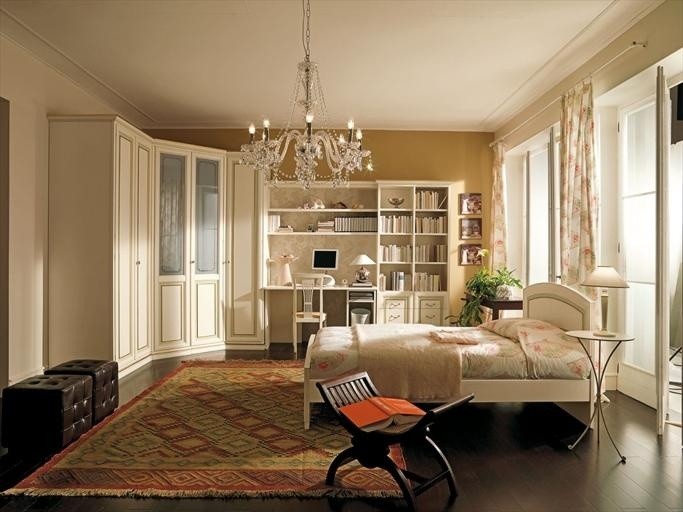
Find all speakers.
<box><xmin>342</xmin><ymin>279</ymin><xmax>348</xmax><ymax>286</ymax></box>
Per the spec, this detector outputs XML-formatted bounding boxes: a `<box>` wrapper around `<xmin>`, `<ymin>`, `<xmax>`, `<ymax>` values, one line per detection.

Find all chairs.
<box><xmin>289</xmin><ymin>274</ymin><xmax>327</xmax><ymax>352</ymax></box>
<box><xmin>317</xmin><ymin>371</ymin><xmax>474</xmax><ymax>504</ymax></box>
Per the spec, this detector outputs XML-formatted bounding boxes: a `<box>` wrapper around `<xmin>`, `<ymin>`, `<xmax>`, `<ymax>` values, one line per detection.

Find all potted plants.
<box><xmin>447</xmin><ymin>270</ymin><xmax>524</xmax><ymax>325</ymax></box>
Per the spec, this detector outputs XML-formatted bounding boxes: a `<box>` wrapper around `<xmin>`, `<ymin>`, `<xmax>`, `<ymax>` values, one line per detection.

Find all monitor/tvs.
<box><xmin>311</xmin><ymin>249</ymin><xmax>338</xmax><ymax>274</ymax></box>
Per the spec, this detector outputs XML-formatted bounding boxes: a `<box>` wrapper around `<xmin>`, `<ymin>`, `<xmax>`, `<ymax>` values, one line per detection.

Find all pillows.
<box><xmin>478</xmin><ymin>317</ymin><xmax>557</xmax><ymax>343</ymax></box>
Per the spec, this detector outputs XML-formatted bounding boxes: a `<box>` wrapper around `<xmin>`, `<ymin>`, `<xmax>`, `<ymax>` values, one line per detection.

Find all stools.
<box><xmin>2</xmin><ymin>372</ymin><xmax>91</xmax><ymax>451</ymax></box>
<box><xmin>45</xmin><ymin>359</ymin><xmax>123</xmax><ymax>421</ymax></box>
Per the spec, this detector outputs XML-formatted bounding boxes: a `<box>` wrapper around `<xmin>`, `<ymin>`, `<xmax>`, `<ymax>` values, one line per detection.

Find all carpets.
<box><xmin>0</xmin><ymin>358</ymin><xmax>414</xmax><ymax>498</ymax></box>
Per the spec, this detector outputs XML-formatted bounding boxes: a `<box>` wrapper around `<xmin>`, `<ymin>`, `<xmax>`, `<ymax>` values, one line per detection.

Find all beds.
<box><xmin>304</xmin><ymin>281</ymin><xmax>602</xmax><ymax>433</ymax></box>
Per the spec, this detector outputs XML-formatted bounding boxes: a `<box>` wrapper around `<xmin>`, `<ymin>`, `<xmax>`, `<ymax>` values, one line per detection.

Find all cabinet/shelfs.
<box><xmin>150</xmin><ymin>137</ymin><xmax>224</xmax><ymax>360</ymax></box>
<box><xmin>48</xmin><ymin>115</ymin><xmax>150</xmax><ymax>378</ymax></box>
<box><xmin>224</xmin><ymin>147</ymin><xmax>269</xmax><ymax>350</ymax></box>
<box><xmin>379</xmin><ymin>180</ymin><xmax>453</xmax><ymax>327</ymax></box>
<box><xmin>264</xmin><ymin>181</ymin><xmax>381</xmax><ymax>343</ymax></box>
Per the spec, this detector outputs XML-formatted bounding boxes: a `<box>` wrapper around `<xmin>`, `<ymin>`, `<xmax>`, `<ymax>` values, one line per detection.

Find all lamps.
<box><xmin>581</xmin><ymin>264</ymin><xmax>631</xmax><ymax>338</ymax></box>
<box><xmin>234</xmin><ymin>0</ymin><xmax>374</xmax><ymax>187</ymax></box>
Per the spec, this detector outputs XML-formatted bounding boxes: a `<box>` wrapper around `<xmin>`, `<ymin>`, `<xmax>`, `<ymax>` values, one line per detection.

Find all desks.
<box><xmin>462</xmin><ymin>290</ymin><xmax>522</xmax><ymax>320</ymax></box>
<box><xmin>564</xmin><ymin>331</ymin><xmax>635</xmax><ymax>460</ymax></box>
<box><xmin>266</xmin><ymin>283</ymin><xmax>376</xmax><ymax>328</ymax></box>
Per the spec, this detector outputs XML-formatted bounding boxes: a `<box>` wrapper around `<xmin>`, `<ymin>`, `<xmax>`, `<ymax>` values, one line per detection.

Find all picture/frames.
<box><xmin>459</xmin><ymin>217</ymin><xmax>483</xmax><ymax>241</ymax></box>
<box><xmin>459</xmin><ymin>243</ymin><xmax>482</xmax><ymax>264</ymax></box>
<box><xmin>459</xmin><ymin>193</ymin><xmax>483</xmax><ymax>214</ymax></box>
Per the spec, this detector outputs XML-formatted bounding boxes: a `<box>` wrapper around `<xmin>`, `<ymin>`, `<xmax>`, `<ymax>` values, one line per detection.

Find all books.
<box><xmin>351</xmin><ymin>282</ymin><xmax>372</xmax><ymax>287</ymax></box>
<box><xmin>380</xmin><ymin>214</ymin><xmax>412</xmax><ymax>233</ymax></box>
<box><xmin>416</xmin><ymin>272</ymin><xmax>442</xmax><ymax>292</ymax></box>
<box><xmin>334</xmin><ymin>216</ymin><xmax>378</xmax><ymax>232</ymax></box>
<box><xmin>317</xmin><ymin>220</ymin><xmax>335</xmax><ymax>232</ymax></box>
<box><xmin>415</xmin><ymin>242</ymin><xmax>447</xmax><ymax>262</ymax></box>
<box><xmin>416</xmin><ymin>214</ymin><xmax>446</xmax><ymax>234</ymax></box>
<box><xmin>417</xmin><ymin>190</ymin><xmax>447</xmax><ymax>209</ymax></box>
<box><xmin>381</xmin><ymin>270</ymin><xmax>411</xmax><ymax>292</ymax></box>
<box><xmin>382</xmin><ymin>244</ymin><xmax>412</xmax><ymax>263</ymax></box>
<box><xmin>340</xmin><ymin>396</ymin><xmax>426</xmax><ymax>432</ymax></box>
<box><xmin>269</xmin><ymin>215</ymin><xmax>280</xmax><ymax>232</ymax></box>
<box><xmin>380</xmin><ymin>422</ymin><xmax>418</xmax><ymax>435</ymax></box>
